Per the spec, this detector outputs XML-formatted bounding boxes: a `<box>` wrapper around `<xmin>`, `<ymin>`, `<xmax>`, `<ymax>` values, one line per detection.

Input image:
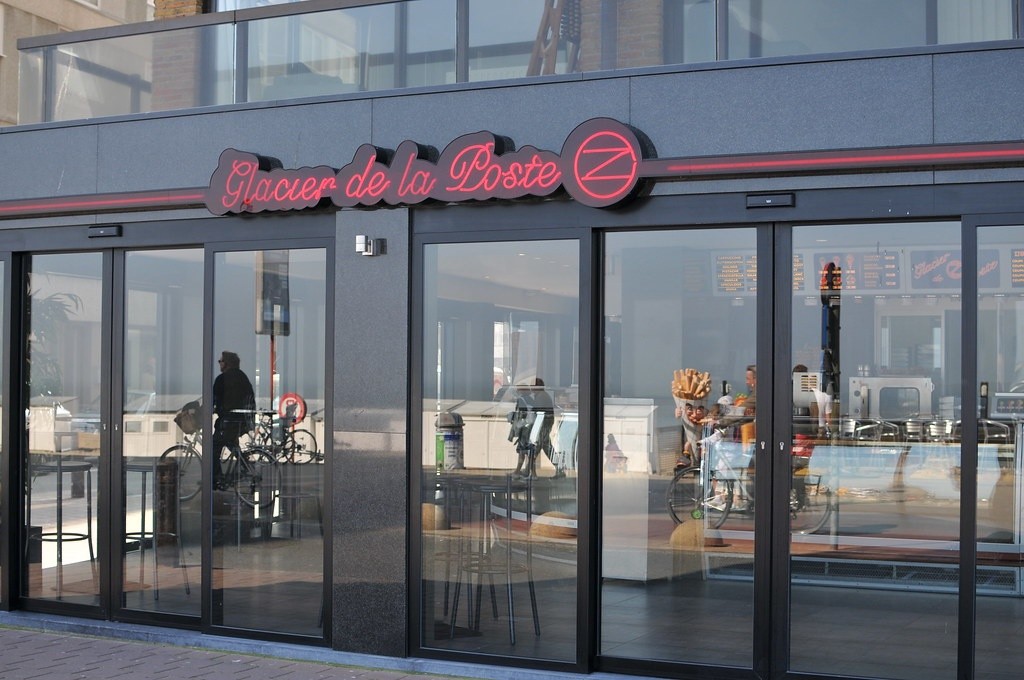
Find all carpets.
<box><xmin>51</xmin><ymin>577</ymin><xmax>150</xmax><ymax>596</ymax></box>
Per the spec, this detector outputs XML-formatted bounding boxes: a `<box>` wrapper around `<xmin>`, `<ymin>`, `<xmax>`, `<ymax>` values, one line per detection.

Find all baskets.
<box><xmin>173</xmin><ymin>409</ymin><xmax>202</xmax><ymax>434</ymax></box>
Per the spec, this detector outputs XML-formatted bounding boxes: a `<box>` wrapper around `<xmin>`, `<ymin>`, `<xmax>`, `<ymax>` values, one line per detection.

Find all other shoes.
<box><xmin>213</xmin><ymin>479</ymin><xmax>222</xmax><ymax>490</ymax></box>
<box><xmin>549</xmin><ymin>473</ymin><xmax>566</xmax><ymax>480</ymax></box>
<box><xmin>524</xmin><ymin>475</ymin><xmax>538</xmax><ymax>480</ymax></box>
<box><xmin>508</xmin><ymin>473</ymin><xmax>520</xmax><ymax>478</ymax></box>
<box><xmin>244</xmin><ymin>465</ymin><xmax>255</xmax><ymax>474</ymax></box>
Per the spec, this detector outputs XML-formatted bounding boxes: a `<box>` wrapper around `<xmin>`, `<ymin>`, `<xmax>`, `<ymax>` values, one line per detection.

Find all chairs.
<box><xmin>277</xmin><ymin>449</ymin><xmax>322</xmax><ymax>543</ymax></box>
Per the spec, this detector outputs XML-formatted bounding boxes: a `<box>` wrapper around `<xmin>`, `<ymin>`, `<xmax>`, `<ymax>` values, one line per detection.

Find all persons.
<box><xmin>507</xmin><ymin>379</ymin><xmax>566</xmax><ymax>481</ymax></box>
<box><xmin>603</xmin><ymin>434</ymin><xmax>620</xmax><ymax>473</ymax></box>
<box><xmin>212</xmin><ymin>350</ymin><xmax>256</xmax><ymax>491</ymax></box>
<box><xmin>791</xmin><ymin>364</ymin><xmax>832</xmax><ymax>507</ymax></box>
<box><xmin>707</xmin><ymin>365</ymin><xmax>757</xmax><ymax>431</ymax></box>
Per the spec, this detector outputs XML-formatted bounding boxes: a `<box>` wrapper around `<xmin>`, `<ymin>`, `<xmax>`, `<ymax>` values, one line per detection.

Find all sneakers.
<box><xmin>724</xmin><ymin>500</ymin><xmax>748</xmax><ymax>511</ymax></box>
<box><xmin>704</xmin><ymin>497</ymin><xmax>727</xmax><ymax>511</ymax></box>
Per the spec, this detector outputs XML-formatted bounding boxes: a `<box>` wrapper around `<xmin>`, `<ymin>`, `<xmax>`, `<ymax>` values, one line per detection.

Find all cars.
<box><xmin>72</xmin><ymin>389</ymin><xmax>156</xmax><ymax>434</ymax></box>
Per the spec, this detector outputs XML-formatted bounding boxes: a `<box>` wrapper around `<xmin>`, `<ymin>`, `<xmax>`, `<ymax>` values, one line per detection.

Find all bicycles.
<box><xmin>160</xmin><ymin>409</ymin><xmax>318</xmax><ymax>508</ymax></box>
<box><xmin>665</xmin><ymin>417</ymin><xmax>831</xmax><ymax>535</ymax></box>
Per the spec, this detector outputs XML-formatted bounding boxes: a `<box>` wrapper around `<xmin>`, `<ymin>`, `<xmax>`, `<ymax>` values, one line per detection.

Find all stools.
<box><xmin>122</xmin><ymin>417</ymin><xmax>191</xmax><ymax>599</ymax></box>
<box><xmin>451</xmin><ymin>444</ymin><xmax>542</xmax><ymax>646</ymax></box>
<box><xmin>29</xmin><ymin>404</ymin><xmax>96</xmax><ymax>599</ymax></box>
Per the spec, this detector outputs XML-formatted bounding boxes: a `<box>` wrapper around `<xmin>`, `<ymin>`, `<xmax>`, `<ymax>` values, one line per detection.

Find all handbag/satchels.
<box><xmin>515</xmin><ymin>397</ymin><xmax>535</xmax><ymax>427</ymax></box>
